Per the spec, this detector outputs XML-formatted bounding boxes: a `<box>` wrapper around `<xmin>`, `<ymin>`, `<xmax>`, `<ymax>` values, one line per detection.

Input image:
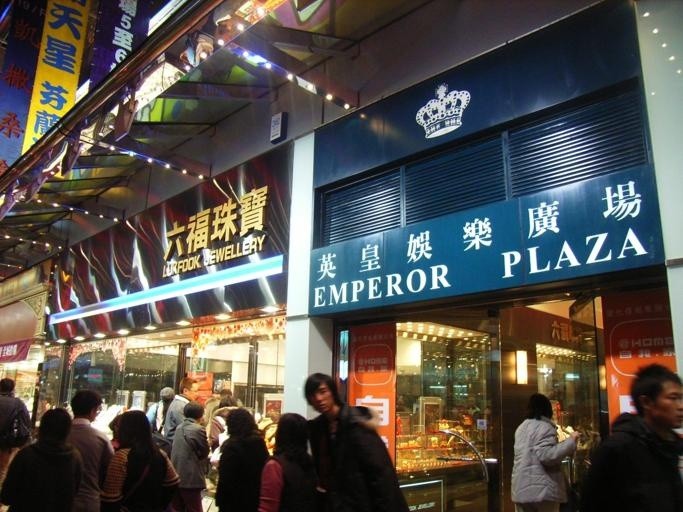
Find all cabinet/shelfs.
<box><xmin>395</xmin><ymin>428</ymin><xmax>490</xmax><ymax>512</ymax></box>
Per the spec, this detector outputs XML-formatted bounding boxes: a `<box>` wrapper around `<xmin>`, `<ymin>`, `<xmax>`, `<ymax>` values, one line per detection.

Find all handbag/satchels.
<box><xmin>152</xmin><ymin>432</ymin><xmax>171</xmax><ymax>459</ymax></box>
<box><xmin>2</xmin><ymin>407</ymin><xmax>30</xmax><ymax>447</ymax></box>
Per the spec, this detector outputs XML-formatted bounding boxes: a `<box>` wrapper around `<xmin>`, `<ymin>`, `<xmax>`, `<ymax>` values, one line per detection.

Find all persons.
<box><xmin>146</xmin><ymin>378</ymin><xmax>251</xmax><ymax>511</ymax></box>
<box><xmin>509</xmin><ymin>393</ymin><xmax>582</xmax><ymax>512</ymax></box>
<box><xmin>215</xmin><ymin>372</ymin><xmax>409</xmax><ymax>511</ymax></box>
<box><xmin>1</xmin><ymin>378</ymin><xmax>183</xmax><ymax>512</ymax></box>
<box><xmin>584</xmin><ymin>361</ymin><xmax>683</xmax><ymax>512</ymax></box>
